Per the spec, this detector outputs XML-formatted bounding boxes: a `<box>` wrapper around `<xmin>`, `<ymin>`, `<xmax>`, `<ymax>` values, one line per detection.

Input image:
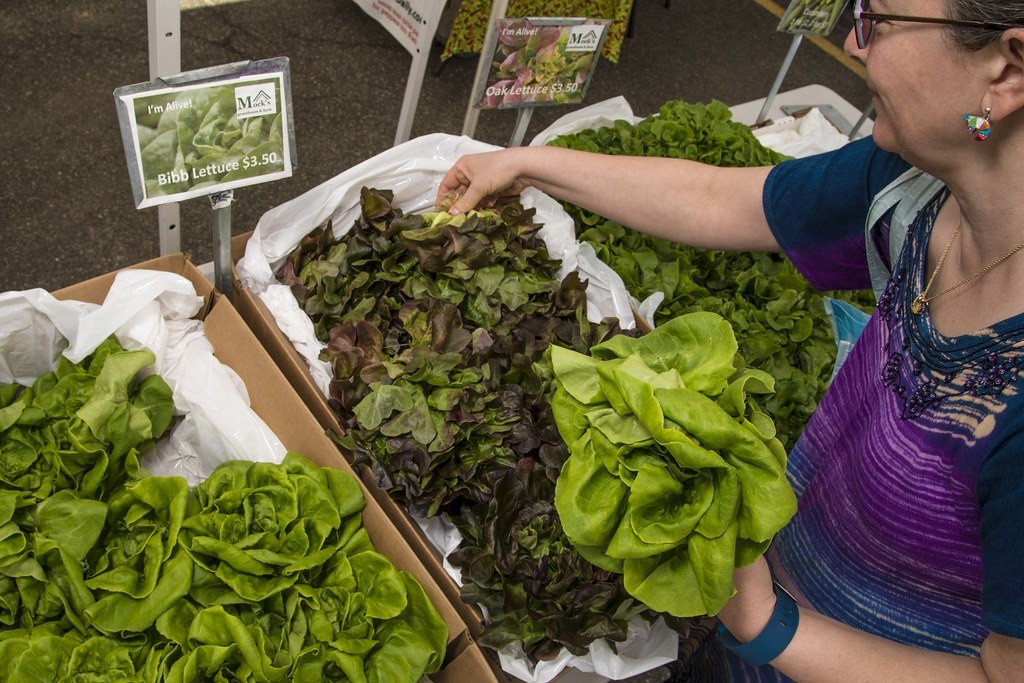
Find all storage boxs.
<box><xmin>0</xmin><ymin>86</ymin><xmax>876</xmax><ymax>683</ymax></box>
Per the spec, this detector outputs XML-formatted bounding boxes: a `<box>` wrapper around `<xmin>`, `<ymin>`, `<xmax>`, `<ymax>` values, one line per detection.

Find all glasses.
<box><xmin>852</xmin><ymin>0</ymin><xmax>1024</xmax><ymax>49</ymax></box>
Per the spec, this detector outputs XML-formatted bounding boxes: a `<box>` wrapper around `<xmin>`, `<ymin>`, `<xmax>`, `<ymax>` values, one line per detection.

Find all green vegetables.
<box><xmin>133</xmin><ymin>78</ymin><xmax>284</xmax><ymax>197</ymax></box>
<box><xmin>0</xmin><ymin>98</ymin><xmax>880</xmax><ymax>683</ymax></box>
<box><xmin>477</xmin><ymin>20</ymin><xmax>597</xmax><ymax>103</ymax></box>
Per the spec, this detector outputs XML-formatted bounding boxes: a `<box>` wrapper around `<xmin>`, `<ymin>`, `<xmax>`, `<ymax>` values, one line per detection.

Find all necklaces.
<box><xmin>911</xmin><ymin>221</ymin><xmax>1024</xmax><ymax>315</ymax></box>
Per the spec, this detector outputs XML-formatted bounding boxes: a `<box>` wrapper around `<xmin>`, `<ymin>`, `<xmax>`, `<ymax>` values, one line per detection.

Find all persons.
<box><xmin>435</xmin><ymin>2</ymin><xmax>1024</xmax><ymax>683</ymax></box>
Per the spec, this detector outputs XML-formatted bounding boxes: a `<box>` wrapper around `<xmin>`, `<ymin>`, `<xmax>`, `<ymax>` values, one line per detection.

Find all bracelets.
<box><xmin>717</xmin><ymin>581</ymin><xmax>800</xmax><ymax>668</ymax></box>
